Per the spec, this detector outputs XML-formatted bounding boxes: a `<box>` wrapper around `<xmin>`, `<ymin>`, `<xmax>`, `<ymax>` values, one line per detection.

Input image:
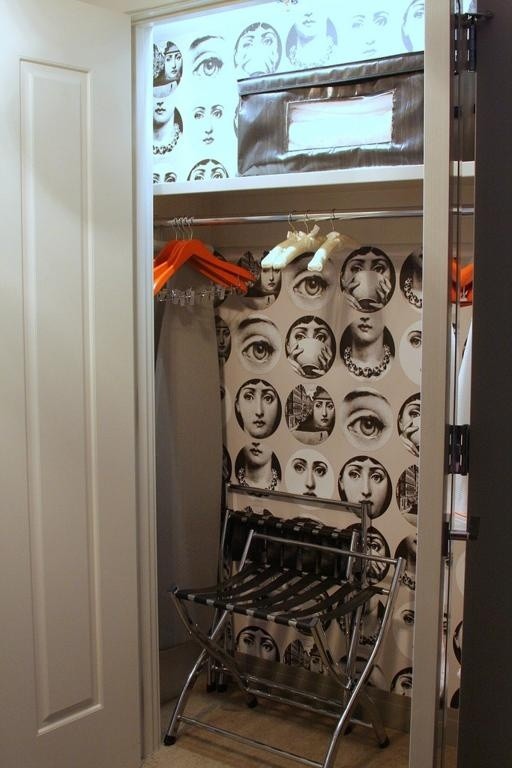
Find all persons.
<box><xmin>214</xmin><ymin>246</ymin><xmax>462</xmax><ymax>711</ymax></box>
<box><xmin>153</xmin><ymin>0</ymin><xmax>477</xmax><ymax>185</ymax></box>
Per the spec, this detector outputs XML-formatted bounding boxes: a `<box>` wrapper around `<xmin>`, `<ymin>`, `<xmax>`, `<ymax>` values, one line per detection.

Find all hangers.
<box><xmin>144</xmin><ymin>205</ymin><xmax>365</xmax><ymax>303</ymax></box>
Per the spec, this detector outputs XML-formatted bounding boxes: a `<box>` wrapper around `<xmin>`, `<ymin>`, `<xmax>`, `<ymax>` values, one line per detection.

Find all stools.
<box><xmin>155</xmin><ymin>524</ymin><xmax>410</xmax><ymax>768</ymax></box>
<box><xmin>197</xmin><ymin>477</ymin><xmax>381</xmax><ymax>737</ymax></box>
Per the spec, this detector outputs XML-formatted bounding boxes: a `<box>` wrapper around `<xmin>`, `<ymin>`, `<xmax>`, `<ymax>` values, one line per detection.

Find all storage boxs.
<box><xmin>231</xmin><ymin>44</ymin><xmax>475</xmax><ymax>180</ymax></box>
